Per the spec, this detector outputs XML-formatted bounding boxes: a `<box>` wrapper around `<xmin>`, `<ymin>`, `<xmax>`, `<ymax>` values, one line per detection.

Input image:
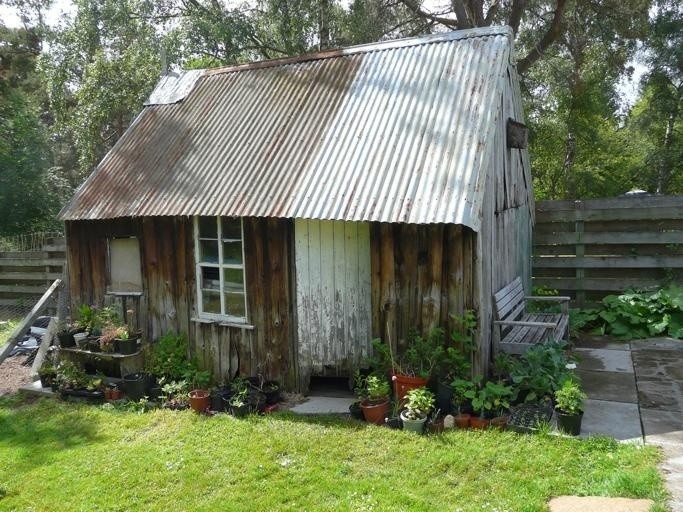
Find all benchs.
<box><xmin>488</xmin><ymin>274</ymin><xmax>575</xmax><ymax>374</ymax></box>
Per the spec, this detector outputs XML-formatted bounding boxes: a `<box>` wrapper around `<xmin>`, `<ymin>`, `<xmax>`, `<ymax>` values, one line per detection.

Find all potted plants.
<box><xmin>24</xmin><ymin>299</ymin><xmax>285</xmax><ymax>421</ymax></box>
<box><xmin>341</xmin><ymin>299</ymin><xmax>588</xmax><ymax>439</ymax></box>
